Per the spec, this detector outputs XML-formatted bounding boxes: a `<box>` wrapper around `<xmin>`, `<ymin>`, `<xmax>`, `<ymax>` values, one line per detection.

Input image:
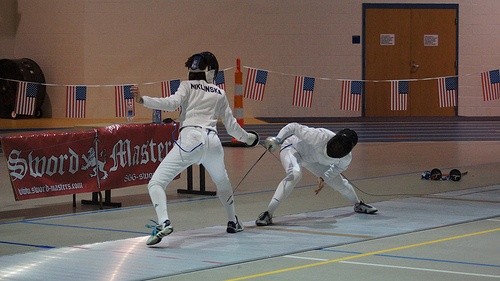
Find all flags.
<box><xmin>161</xmin><ymin>79</ymin><xmax>181</xmax><ymax>112</ymax></box>
<box><xmin>16</xmin><ymin>81</ymin><xmax>39</xmax><ymax>115</ymax></box>
<box><xmin>65</xmin><ymin>85</ymin><xmax>87</xmax><ymax>118</ymax></box>
<box><xmin>291</xmin><ymin>75</ymin><xmax>315</xmax><ymax>108</ymax></box>
<box><xmin>438</xmin><ymin>77</ymin><xmax>458</xmax><ymax>107</ymax></box>
<box><xmin>114</xmin><ymin>85</ymin><xmax>136</xmax><ymax>118</ymax></box>
<box><xmin>212</xmin><ymin>71</ymin><xmax>226</xmax><ymax>92</ymax></box>
<box><xmin>243</xmin><ymin>68</ymin><xmax>268</xmax><ymax>101</ymax></box>
<box><xmin>390</xmin><ymin>81</ymin><xmax>408</xmax><ymax>111</ymax></box>
<box><xmin>480</xmin><ymin>70</ymin><xmax>500</xmax><ymax>101</ymax></box>
<box><xmin>339</xmin><ymin>80</ymin><xmax>363</xmax><ymax>111</ymax></box>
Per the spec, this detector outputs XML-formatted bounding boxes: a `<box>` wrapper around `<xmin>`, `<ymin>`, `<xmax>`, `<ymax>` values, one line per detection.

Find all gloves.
<box><xmin>314</xmin><ymin>177</ymin><xmax>324</xmax><ymax>195</ymax></box>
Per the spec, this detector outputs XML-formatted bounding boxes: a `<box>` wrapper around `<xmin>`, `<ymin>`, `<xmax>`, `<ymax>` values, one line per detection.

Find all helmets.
<box><xmin>185</xmin><ymin>52</ymin><xmax>219</xmax><ymax>84</ymax></box>
<box><xmin>327</xmin><ymin>128</ymin><xmax>358</xmax><ymax>159</ymax></box>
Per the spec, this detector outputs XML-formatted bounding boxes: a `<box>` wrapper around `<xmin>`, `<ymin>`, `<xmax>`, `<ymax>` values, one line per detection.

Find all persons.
<box><xmin>254</xmin><ymin>122</ymin><xmax>379</xmax><ymax>225</ymax></box>
<box><xmin>130</xmin><ymin>51</ymin><xmax>260</xmax><ymax>246</ymax></box>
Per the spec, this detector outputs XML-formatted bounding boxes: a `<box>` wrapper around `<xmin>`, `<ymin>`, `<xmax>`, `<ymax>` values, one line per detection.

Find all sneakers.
<box><xmin>354</xmin><ymin>201</ymin><xmax>378</xmax><ymax>214</ymax></box>
<box><xmin>146</xmin><ymin>218</ymin><xmax>174</xmax><ymax>246</ymax></box>
<box><xmin>226</xmin><ymin>214</ymin><xmax>244</xmax><ymax>233</ymax></box>
<box><xmin>255</xmin><ymin>210</ymin><xmax>272</xmax><ymax>226</ymax></box>
